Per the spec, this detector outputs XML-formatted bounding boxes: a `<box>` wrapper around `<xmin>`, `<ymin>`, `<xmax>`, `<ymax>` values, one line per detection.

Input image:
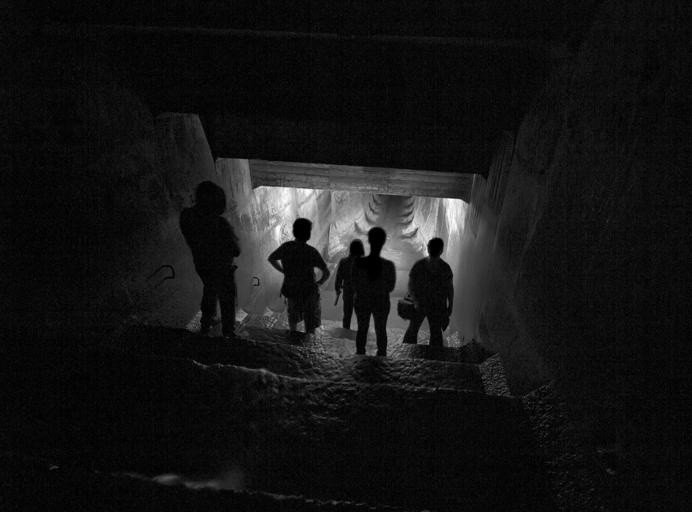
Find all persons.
<box><xmin>335</xmin><ymin>239</ymin><xmax>364</xmax><ymax>328</ymax></box>
<box><xmin>350</xmin><ymin>226</ymin><xmax>396</xmax><ymax>356</ymax></box>
<box><xmin>402</xmin><ymin>237</ymin><xmax>453</xmax><ymax>347</ymax></box>
<box><xmin>267</xmin><ymin>218</ymin><xmax>329</xmax><ymax>334</ymax></box>
<box><xmin>179</xmin><ymin>181</ymin><xmax>241</xmax><ymax>337</ymax></box>
